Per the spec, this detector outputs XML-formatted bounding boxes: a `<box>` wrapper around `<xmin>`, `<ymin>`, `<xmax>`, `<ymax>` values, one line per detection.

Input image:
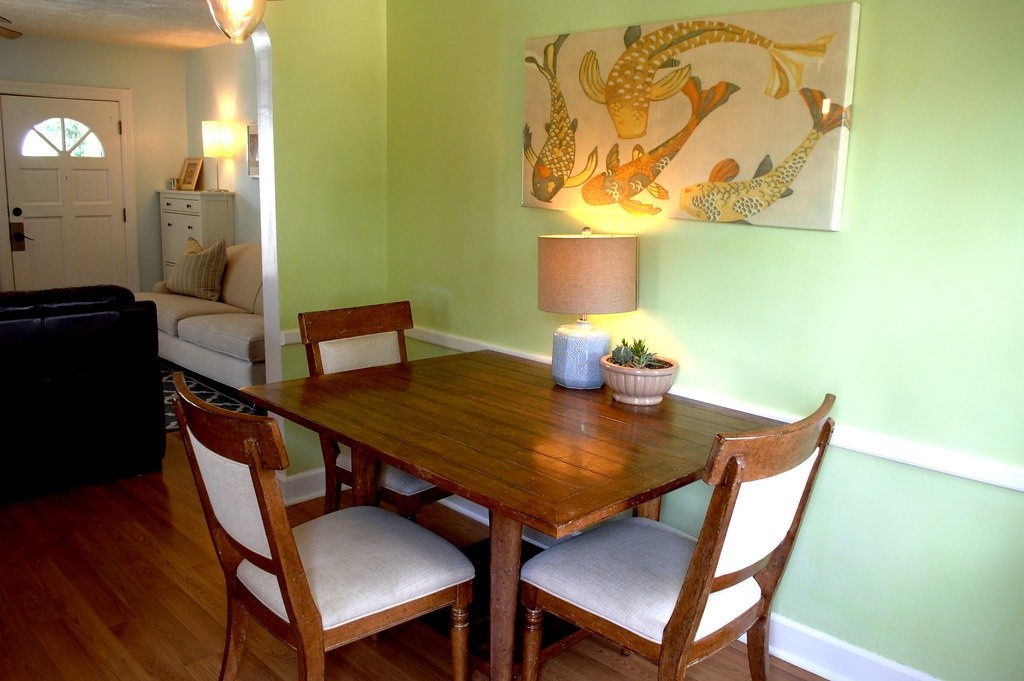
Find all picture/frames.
<box><xmin>176</xmin><ymin>157</ymin><xmax>203</xmax><ymax>190</ymax></box>
<box><xmin>247</xmin><ymin>125</ymin><xmax>259</xmax><ymax>178</ymax></box>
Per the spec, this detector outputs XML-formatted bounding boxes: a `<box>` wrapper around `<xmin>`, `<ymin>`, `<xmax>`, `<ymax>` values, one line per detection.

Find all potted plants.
<box><xmin>600</xmin><ymin>338</ymin><xmax>678</xmax><ymax>406</ymax></box>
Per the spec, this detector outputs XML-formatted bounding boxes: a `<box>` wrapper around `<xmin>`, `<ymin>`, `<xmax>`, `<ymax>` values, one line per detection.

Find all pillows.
<box><xmin>165</xmin><ymin>236</ymin><xmax>227</xmax><ymax>300</ymax></box>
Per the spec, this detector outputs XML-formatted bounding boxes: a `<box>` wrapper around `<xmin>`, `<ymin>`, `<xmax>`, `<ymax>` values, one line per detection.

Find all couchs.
<box><xmin>0</xmin><ymin>286</ymin><xmax>166</xmax><ymax>503</ymax></box>
<box><xmin>135</xmin><ymin>241</ymin><xmax>266</xmax><ymax>415</ymax></box>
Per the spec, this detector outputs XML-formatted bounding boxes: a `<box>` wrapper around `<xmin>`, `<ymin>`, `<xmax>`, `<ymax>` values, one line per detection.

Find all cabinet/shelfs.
<box><xmin>156</xmin><ymin>189</ymin><xmax>236</xmax><ymax>281</ymax></box>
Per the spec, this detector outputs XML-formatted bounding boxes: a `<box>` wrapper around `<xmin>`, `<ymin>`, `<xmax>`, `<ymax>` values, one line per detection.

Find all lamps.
<box><xmin>538</xmin><ymin>227</ymin><xmax>639</xmax><ymax>390</ymax></box>
<box><xmin>205</xmin><ymin>0</ymin><xmax>266</xmax><ymax>44</ymax></box>
<box><xmin>201</xmin><ymin>121</ymin><xmax>235</xmax><ymax>193</ymax></box>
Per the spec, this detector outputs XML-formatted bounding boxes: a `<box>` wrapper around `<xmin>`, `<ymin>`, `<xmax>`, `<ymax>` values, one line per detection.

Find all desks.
<box><xmin>240</xmin><ymin>350</ymin><xmax>792</xmax><ymax>681</ymax></box>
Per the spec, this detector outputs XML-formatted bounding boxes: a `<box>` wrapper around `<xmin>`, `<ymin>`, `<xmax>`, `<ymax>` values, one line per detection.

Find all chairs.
<box><xmin>171</xmin><ymin>371</ymin><xmax>477</xmax><ymax>681</ymax></box>
<box><xmin>518</xmin><ymin>395</ymin><xmax>838</xmax><ymax>681</ymax></box>
<box><xmin>299</xmin><ymin>299</ymin><xmax>456</xmax><ymax>525</ymax></box>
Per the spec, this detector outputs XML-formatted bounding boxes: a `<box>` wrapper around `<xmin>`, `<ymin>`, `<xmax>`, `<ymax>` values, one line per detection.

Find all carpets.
<box><xmin>161</xmin><ymin>366</ymin><xmax>264</xmax><ymax>430</ymax></box>
